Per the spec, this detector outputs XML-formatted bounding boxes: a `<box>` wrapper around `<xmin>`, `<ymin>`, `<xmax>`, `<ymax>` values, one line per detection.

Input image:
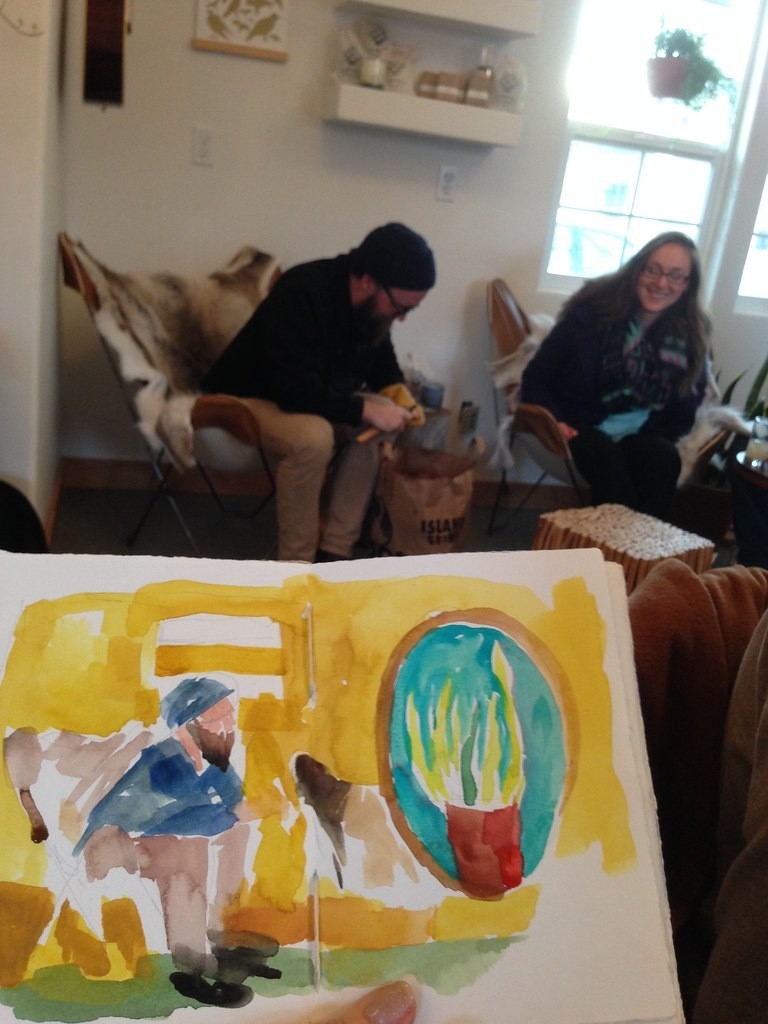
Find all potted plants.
<box><xmin>668</xmin><ymin>352</ymin><xmax>768</xmax><ymax>540</ymax></box>
<box><xmin>644</xmin><ymin>28</ymin><xmax>738</xmax><ymax>112</ymax></box>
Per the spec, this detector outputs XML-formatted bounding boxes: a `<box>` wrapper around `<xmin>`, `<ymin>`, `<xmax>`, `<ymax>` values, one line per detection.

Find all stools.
<box><xmin>532</xmin><ymin>503</ymin><xmax>718</xmax><ymax>604</ymax></box>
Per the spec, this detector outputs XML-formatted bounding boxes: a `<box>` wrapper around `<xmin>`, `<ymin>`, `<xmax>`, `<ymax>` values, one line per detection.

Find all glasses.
<box><xmin>383</xmin><ymin>287</ymin><xmax>415</xmax><ymax>317</ymax></box>
<box><xmin>640</xmin><ymin>260</ymin><xmax>693</xmax><ymax>286</ymax></box>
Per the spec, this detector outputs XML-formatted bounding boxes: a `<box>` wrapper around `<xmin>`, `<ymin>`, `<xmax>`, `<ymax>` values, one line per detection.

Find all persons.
<box><xmin>201</xmin><ymin>223</ymin><xmax>435</xmax><ymax>562</ymax></box>
<box><xmin>516</xmin><ymin>229</ymin><xmax>710</xmax><ymax>521</ymax></box>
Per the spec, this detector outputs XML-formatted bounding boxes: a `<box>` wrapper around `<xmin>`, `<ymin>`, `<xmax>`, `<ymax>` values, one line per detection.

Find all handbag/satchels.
<box><xmin>381</xmin><ymin>434</ymin><xmax>485</xmax><ymax>555</ymax></box>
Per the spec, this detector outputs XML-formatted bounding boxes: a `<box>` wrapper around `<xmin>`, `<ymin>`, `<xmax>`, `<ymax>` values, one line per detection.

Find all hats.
<box><xmin>358</xmin><ymin>222</ymin><xmax>436</xmax><ymax>291</ymax></box>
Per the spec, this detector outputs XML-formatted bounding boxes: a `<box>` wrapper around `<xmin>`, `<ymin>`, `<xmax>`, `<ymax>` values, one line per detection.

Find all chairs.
<box><xmin>483</xmin><ymin>278</ymin><xmax>593</xmax><ymax>536</ymax></box>
<box><xmin>60</xmin><ymin>231</ymin><xmax>276</xmax><ymax>561</ymax></box>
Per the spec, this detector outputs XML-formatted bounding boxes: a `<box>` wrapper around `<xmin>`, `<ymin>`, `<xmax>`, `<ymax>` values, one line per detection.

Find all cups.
<box><xmin>745</xmin><ymin>416</ymin><xmax>768</xmax><ymax>469</ymax></box>
